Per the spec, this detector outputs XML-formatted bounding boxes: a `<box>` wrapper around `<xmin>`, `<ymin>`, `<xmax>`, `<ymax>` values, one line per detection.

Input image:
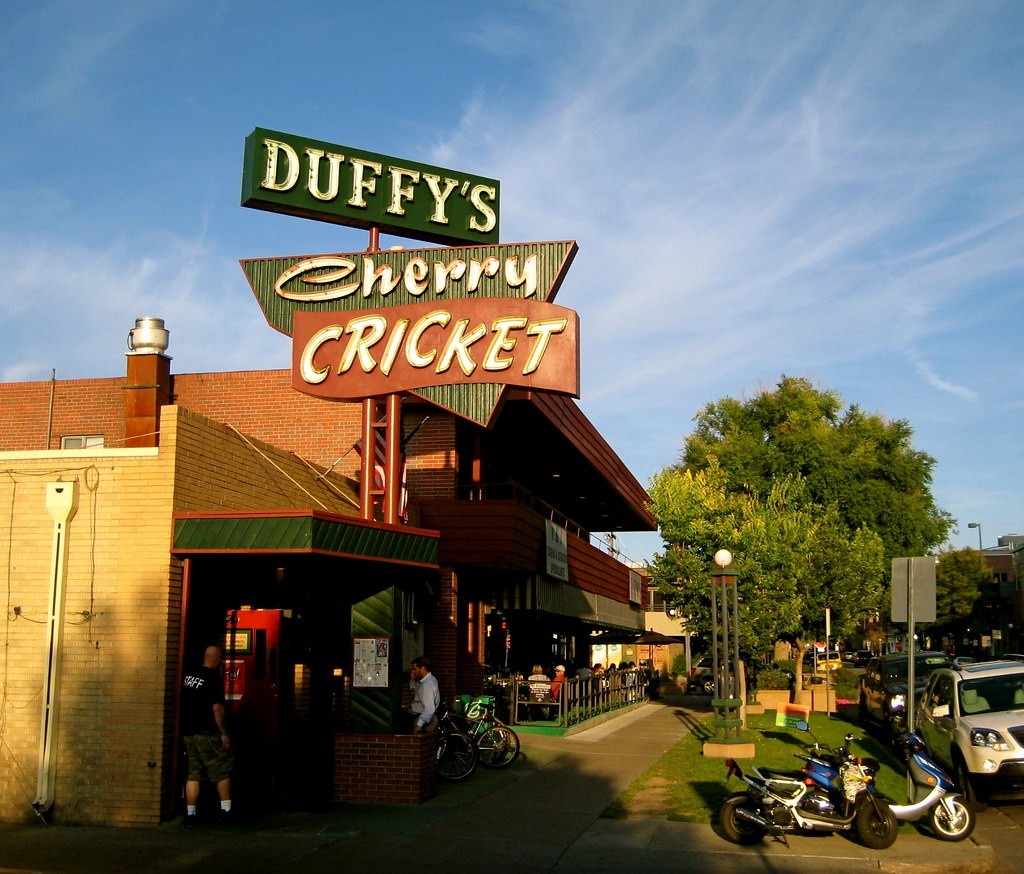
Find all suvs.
<box><xmin>856</xmin><ymin>651</ymin><xmax>966</xmax><ymax>752</ymax></box>
<box><xmin>914</xmin><ymin>651</ymin><xmax>1024</xmax><ymax>812</ymax></box>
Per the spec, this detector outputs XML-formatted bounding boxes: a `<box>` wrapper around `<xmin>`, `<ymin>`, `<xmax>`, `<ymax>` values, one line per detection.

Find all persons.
<box><xmin>549</xmin><ymin>662</ymin><xmax>646</xmax><ymax>703</ymax></box>
<box><xmin>528</xmin><ymin>665</ymin><xmax>551</xmax><ymax>719</ymax></box>
<box><xmin>179</xmin><ymin>646</ymin><xmax>232</xmax><ymax>828</ymax></box>
<box><xmin>408</xmin><ymin>657</ymin><xmax>440</xmax><ymax>733</ymax></box>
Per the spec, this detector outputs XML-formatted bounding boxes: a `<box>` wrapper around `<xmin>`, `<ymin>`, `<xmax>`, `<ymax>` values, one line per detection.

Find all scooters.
<box><xmin>795</xmin><ymin>715</ymin><xmax>979</xmax><ymax>841</ymax></box>
<box><xmin>704</xmin><ymin>719</ymin><xmax>899</xmax><ymax>850</ymax></box>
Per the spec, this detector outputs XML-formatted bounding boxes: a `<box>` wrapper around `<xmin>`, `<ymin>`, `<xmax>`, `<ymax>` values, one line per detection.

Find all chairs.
<box><xmin>1014</xmin><ymin>683</ymin><xmax>1024</xmax><ymax>704</ymax></box>
<box><xmin>962</xmin><ymin>690</ymin><xmax>990</xmax><ymax>713</ymax></box>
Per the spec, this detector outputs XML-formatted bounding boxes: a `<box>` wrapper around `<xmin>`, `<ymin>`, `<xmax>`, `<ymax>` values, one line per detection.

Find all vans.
<box><xmin>816</xmin><ymin>651</ymin><xmax>841</xmax><ymax>673</ymax></box>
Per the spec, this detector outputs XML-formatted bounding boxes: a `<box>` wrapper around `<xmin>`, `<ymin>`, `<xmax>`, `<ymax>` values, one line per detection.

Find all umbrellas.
<box><xmin>633</xmin><ymin>631</ymin><xmax>684</xmax><ymax>660</ymax></box>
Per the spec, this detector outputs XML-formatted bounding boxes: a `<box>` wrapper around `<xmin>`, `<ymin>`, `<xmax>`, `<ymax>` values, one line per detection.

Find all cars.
<box><xmin>690</xmin><ymin>656</ymin><xmax>731</xmax><ymax>693</ymax></box>
<box><xmin>954</xmin><ymin>656</ymin><xmax>976</xmax><ymax>670</ymax></box>
<box><xmin>841</xmin><ymin>649</ymin><xmax>875</xmax><ymax>668</ymax></box>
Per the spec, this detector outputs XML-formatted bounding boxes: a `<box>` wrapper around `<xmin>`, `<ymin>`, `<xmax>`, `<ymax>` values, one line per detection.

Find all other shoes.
<box><xmin>216</xmin><ymin>803</ymin><xmax>240</xmax><ymax>825</ymax></box>
<box><xmin>184</xmin><ymin>812</ymin><xmax>200</xmax><ymax>826</ymax></box>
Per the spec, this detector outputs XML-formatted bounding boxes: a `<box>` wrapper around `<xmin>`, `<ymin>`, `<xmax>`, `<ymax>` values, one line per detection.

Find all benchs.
<box><xmin>515</xmin><ymin>681</ymin><xmax>563</xmax><ymax>723</ymax></box>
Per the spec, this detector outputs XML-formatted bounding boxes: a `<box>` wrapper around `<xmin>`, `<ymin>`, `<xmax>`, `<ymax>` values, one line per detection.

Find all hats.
<box><xmin>554</xmin><ymin>665</ymin><xmax>565</xmax><ymax>672</ymax></box>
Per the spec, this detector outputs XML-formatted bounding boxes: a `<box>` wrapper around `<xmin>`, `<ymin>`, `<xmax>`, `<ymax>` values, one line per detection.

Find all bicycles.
<box><xmin>433</xmin><ymin>692</ymin><xmax>520</xmax><ymax>782</ymax></box>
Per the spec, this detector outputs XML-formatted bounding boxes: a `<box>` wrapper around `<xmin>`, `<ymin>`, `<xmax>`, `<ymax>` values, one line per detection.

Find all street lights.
<box><xmin>968</xmin><ymin>521</ymin><xmax>985</xmax><ymax>549</ymax></box>
<box><xmin>716</xmin><ymin>550</ymin><xmax>732</xmax><ymax>737</ymax></box>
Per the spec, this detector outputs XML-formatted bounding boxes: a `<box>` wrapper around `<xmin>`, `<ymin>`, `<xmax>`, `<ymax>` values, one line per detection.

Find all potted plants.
<box><xmin>751</xmin><ymin>669</ymin><xmax>812</xmax><ymax>711</ymax></box>
<box><xmin>829</xmin><ymin>666</ymin><xmax>859</xmax><ymax>711</ymax></box>
<box><xmin>703</xmin><ymin>699</ymin><xmax>756</xmax><ymax>759</ymax></box>
